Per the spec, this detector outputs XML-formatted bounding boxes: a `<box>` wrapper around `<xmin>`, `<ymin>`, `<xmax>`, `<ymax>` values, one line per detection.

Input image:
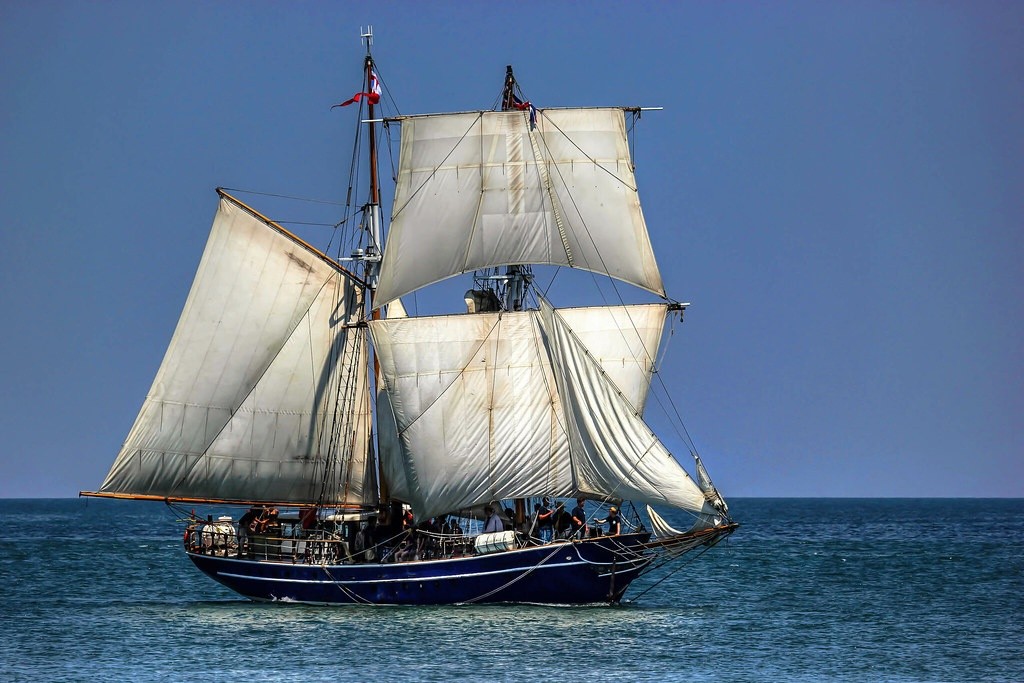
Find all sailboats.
<box><xmin>79</xmin><ymin>23</ymin><xmax>740</xmax><ymax>607</ymax></box>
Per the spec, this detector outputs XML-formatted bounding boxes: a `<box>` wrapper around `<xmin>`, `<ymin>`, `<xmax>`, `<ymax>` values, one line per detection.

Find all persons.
<box><xmin>237</xmin><ymin>498</ymin><xmax>621</xmax><ymax>562</ymax></box>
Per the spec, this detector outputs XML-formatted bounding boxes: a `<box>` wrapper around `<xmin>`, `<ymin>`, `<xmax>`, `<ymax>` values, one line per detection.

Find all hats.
<box><xmin>481</xmin><ymin>506</ymin><xmax>492</xmax><ymax>510</ymax></box>
<box><xmin>556</xmin><ymin>501</ymin><xmax>565</xmax><ymax>508</ymax></box>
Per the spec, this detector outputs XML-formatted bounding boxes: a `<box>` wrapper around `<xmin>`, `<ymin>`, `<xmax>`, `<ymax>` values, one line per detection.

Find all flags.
<box><xmin>330</xmin><ymin>70</ymin><xmax>382</xmax><ymax>112</ymax></box>
